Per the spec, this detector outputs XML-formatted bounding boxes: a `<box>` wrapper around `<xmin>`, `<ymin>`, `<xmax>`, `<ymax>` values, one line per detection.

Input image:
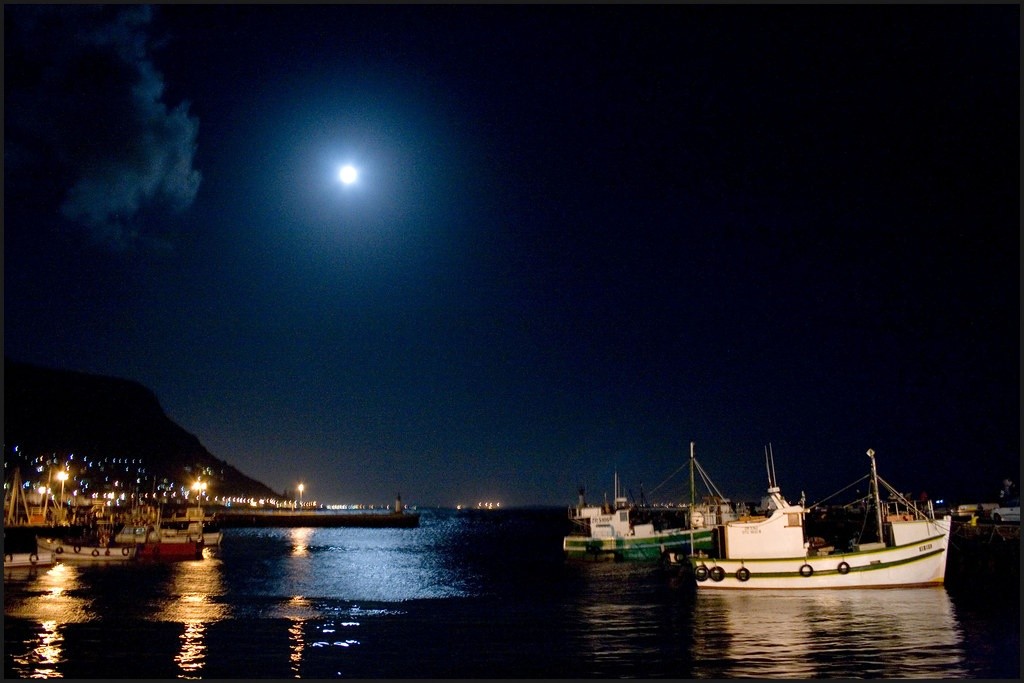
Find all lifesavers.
<box><xmin>837</xmin><ymin>562</ymin><xmax>851</xmax><ymax>575</ymax></box>
<box><xmin>74</xmin><ymin>545</ymin><xmax>81</xmax><ymax>553</ymax></box>
<box><xmin>30</xmin><ymin>553</ymin><xmax>40</xmax><ymax>564</ymax></box>
<box><xmin>4</xmin><ymin>553</ymin><xmax>13</xmax><ymax>564</ymax></box>
<box><xmin>711</xmin><ymin>566</ymin><xmax>725</xmax><ymax>581</ymax></box>
<box><xmin>122</xmin><ymin>546</ymin><xmax>130</xmax><ymax>556</ymax></box>
<box><xmin>92</xmin><ymin>550</ymin><xmax>99</xmax><ymax>556</ymax></box>
<box><xmin>737</xmin><ymin>567</ymin><xmax>751</xmax><ymax>581</ymax></box>
<box><xmin>799</xmin><ymin>564</ymin><xmax>814</xmax><ymax>577</ymax></box>
<box><xmin>695</xmin><ymin>566</ymin><xmax>708</xmax><ymax>582</ymax></box>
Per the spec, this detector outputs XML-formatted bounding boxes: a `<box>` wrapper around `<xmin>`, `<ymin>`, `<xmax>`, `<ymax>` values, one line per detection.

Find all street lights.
<box><xmin>296</xmin><ymin>482</ymin><xmax>305</xmax><ymax>512</ymax></box>
<box><xmin>54</xmin><ymin>470</ymin><xmax>70</xmax><ymax>509</ymax></box>
<box><xmin>191</xmin><ymin>480</ymin><xmax>207</xmax><ymax>509</ymax></box>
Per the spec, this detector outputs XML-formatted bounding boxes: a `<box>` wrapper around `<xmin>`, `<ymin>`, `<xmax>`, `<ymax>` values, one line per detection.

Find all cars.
<box><xmin>990</xmin><ymin>494</ymin><xmax>1020</xmax><ymax>523</ymax></box>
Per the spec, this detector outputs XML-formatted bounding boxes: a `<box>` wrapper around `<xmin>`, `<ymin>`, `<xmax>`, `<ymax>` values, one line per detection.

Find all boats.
<box><xmin>561</xmin><ymin>443</ymin><xmax>770</xmax><ymax>565</ymax></box>
<box><xmin>659</xmin><ymin>442</ymin><xmax>952</xmax><ymax>590</ymax></box>
<box><xmin>0</xmin><ymin>500</ymin><xmax>225</xmax><ymax>581</ymax></box>
